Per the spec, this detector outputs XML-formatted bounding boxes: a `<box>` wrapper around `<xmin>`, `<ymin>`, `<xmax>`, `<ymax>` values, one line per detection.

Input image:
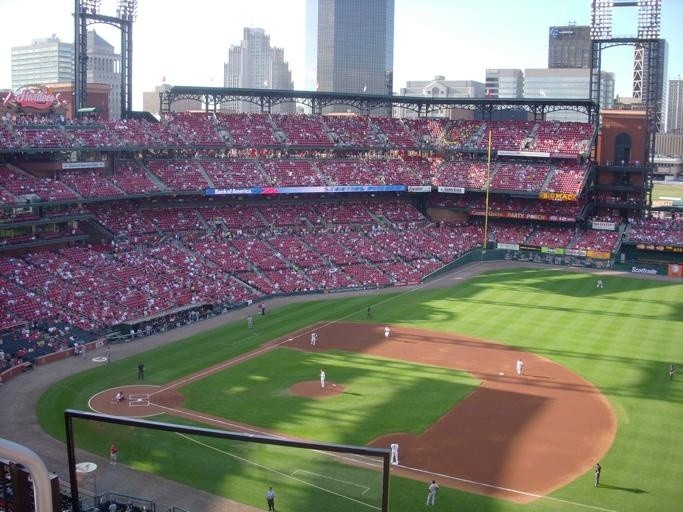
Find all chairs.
<box><xmin>342</xmin><ymin>113</ymin><xmax>660</xmax><ymax>290</ymax></box>
<box><xmin>0</xmin><ymin>98</ymin><xmax>341</xmax><ymax>377</ymax></box>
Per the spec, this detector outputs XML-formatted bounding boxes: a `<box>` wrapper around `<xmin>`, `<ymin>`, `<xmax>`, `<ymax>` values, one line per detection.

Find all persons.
<box><xmin>108</xmin><ymin>501</ymin><xmax>116</xmax><ymax>511</ymax></box>
<box><xmin>515</xmin><ymin>358</ymin><xmax>522</xmax><ymax>374</ymax></box>
<box><xmin>0</xmin><ymin>112</ymin><xmax>681</xmax><ymax>372</ymax></box>
<box><xmin>138</xmin><ymin>361</ymin><xmax>144</xmax><ymax>380</ymax></box>
<box><xmin>426</xmin><ymin>479</ymin><xmax>438</xmax><ymax>504</ymax></box>
<box><xmin>114</xmin><ymin>391</ymin><xmax>125</xmax><ymax>402</ymax></box>
<box><xmin>669</xmin><ymin>364</ymin><xmax>675</xmax><ymax>379</ymax></box>
<box><xmin>266</xmin><ymin>486</ymin><xmax>274</xmax><ymax>510</ymax></box>
<box><xmin>390</xmin><ymin>440</ymin><xmax>398</xmax><ymax>463</ymax></box>
<box><xmin>110</xmin><ymin>443</ymin><xmax>116</xmax><ymax>466</ymax></box>
<box><xmin>594</xmin><ymin>464</ymin><xmax>600</xmax><ymax>485</ymax></box>
<box><xmin>318</xmin><ymin>369</ymin><xmax>325</xmax><ymax>388</ymax></box>
